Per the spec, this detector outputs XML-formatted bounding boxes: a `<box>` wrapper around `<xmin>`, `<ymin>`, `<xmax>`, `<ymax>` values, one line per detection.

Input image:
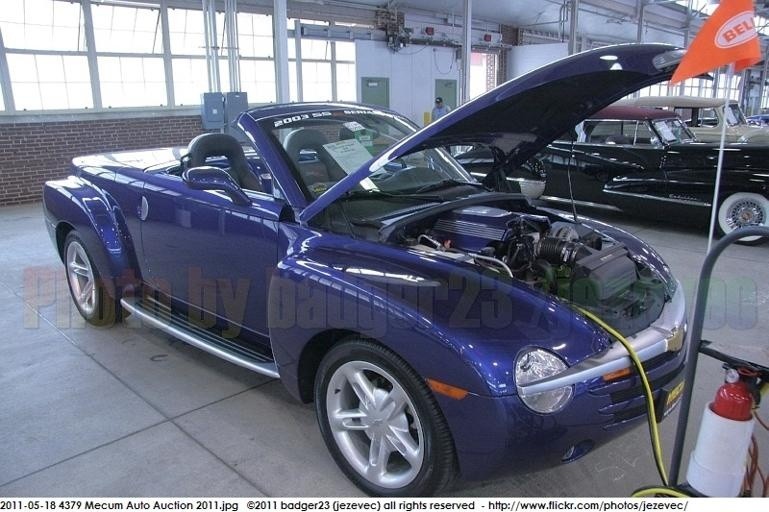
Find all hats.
<box><xmin>435</xmin><ymin>97</ymin><xmax>442</xmax><ymax>103</ymax></box>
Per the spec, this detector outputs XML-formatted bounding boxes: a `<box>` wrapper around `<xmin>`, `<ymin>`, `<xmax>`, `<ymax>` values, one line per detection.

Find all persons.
<box><xmin>432</xmin><ymin>97</ymin><xmax>451</xmax><ymax>154</ymax></box>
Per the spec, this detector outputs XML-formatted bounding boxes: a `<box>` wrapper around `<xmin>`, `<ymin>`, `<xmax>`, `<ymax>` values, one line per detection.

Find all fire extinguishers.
<box><xmin>684</xmin><ymin>362</ymin><xmax>763</xmax><ymax>498</ymax></box>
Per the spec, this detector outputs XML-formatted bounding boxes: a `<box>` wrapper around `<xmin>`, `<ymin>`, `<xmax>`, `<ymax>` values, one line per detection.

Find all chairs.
<box><xmin>283</xmin><ymin>127</ymin><xmax>347</xmax><ymax>197</ymax></box>
<box><xmin>182</xmin><ymin>133</ymin><xmax>262</xmax><ymax>193</ymax></box>
<box><xmin>604</xmin><ymin>134</ymin><xmax>630</xmax><ymax>144</ymax></box>
